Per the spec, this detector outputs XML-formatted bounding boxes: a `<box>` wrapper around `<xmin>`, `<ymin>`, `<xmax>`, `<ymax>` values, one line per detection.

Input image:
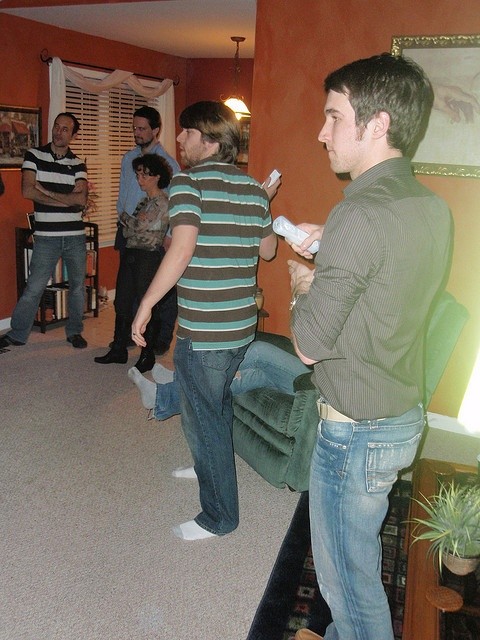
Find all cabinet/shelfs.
<box><xmin>16</xmin><ymin>221</ymin><xmax>100</xmax><ymax>333</ymax></box>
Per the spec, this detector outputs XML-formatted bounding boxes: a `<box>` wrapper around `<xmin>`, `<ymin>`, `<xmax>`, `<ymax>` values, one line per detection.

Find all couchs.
<box><xmin>232</xmin><ymin>289</ymin><xmax>471</xmax><ymax>492</ymax></box>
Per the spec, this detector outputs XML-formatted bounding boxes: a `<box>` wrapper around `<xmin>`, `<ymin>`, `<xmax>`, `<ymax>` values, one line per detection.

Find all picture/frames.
<box><xmin>0</xmin><ymin>104</ymin><xmax>43</xmax><ymax>172</ymax></box>
<box><xmin>390</xmin><ymin>34</ymin><xmax>480</xmax><ymax>179</ymax></box>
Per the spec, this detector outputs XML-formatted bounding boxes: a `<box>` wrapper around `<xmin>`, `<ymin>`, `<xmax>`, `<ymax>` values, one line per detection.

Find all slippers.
<box><xmin>67</xmin><ymin>334</ymin><xmax>87</xmax><ymax>349</ymax></box>
<box><xmin>0</xmin><ymin>335</ymin><xmax>26</xmax><ymax>349</ymax></box>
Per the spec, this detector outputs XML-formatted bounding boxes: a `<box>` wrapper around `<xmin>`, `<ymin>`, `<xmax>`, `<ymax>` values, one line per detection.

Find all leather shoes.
<box><xmin>294</xmin><ymin>628</ymin><xmax>324</xmax><ymax>640</ymax></box>
<box><xmin>94</xmin><ymin>350</ymin><xmax>128</xmax><ymax>364</ymax></box>
<box><xmin>135</xmin><ymin>354</ymin><xmax>155</xmax><ymax>373</ymax></box>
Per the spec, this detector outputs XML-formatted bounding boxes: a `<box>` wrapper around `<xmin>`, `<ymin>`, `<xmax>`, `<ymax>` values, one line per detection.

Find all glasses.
<box><xmin>132</xmin><ymin>126</ymin><xmax>151</xmax><ymax>131</ymax></box>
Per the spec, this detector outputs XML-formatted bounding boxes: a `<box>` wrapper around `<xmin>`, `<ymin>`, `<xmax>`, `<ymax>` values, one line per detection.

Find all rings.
<box><xmin>133</xmin><ymin>332</ymin><xmax>136</xmax><ymax>336</ymax></box>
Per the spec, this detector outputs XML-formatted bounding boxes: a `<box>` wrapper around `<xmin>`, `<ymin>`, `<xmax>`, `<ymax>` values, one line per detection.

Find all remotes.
<box><xmin>272</xmin><ymin>216</ymin><xmax>321</xmax><ymax>254</ymax></box>
<box><xmin>262</xmin><ymin>169</ymin><xmax>282</xmax><ymax>188</ymax></box>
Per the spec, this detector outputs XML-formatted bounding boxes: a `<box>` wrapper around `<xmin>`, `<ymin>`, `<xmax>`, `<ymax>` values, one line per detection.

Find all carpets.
<box><xmin>245</xmin><ymin>479</ymin><xmax>411</xmax><ymax>638</ymax></box>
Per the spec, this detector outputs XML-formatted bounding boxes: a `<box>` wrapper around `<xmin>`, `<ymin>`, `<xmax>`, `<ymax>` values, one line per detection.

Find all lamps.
<box><xmin>219</xmin><ymin>36</ymin><xmax>253</xmax><ymax>120</ymax></box>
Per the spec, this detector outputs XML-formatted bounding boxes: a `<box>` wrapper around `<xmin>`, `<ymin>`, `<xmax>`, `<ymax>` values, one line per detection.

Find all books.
<box><xmin>35</xmin><ymin>288</ymin><xmax>98</xmax><ymax>321</ymax></box>
<box><xmin>23</xmin><ymin>246</ymin><xmax>96</xmax><ymax>286</ymax></box>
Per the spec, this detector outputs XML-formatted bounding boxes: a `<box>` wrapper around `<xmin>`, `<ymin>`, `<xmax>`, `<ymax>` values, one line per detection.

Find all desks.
<box><xmin>400</xmin><ymin>457</ymin><xmax>480</xmax><ymax>639</ymax></box>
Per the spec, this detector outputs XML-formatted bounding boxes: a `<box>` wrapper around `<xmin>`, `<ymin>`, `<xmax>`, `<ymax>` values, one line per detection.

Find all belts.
<box><xmin>316</xmin><ymin>398</ymin><xmax>354</xmax><ymax>422</ymax></box>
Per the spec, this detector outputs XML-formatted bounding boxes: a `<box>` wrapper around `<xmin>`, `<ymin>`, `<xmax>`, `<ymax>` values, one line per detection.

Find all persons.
<box><xmin>0</xmin><ymin>111</ymin><xmax>90</xmax><ymax>349</ymax></box>
<box><xmin>108</xmin><ymin>106</ymin><xmax>181</xmax><ymax>351</ymax></box>
<box><xmin>129</xmin><ymin>102</ymin><xmax>282</xmax><ymax>541</ymax></box>
<box><xmin>93</xmin><ymin>155</ymin><xmax>176</xmax><ymax>375</ymax></box>
<box><xmin>284</xmin><ymin>53</ymin><xmax>454</xmax><ymax>640</ymax></box>
<box><xmin>128</xmin><ymin>340</ymin><xmax>315</xmax><ymax>417</ymax></box>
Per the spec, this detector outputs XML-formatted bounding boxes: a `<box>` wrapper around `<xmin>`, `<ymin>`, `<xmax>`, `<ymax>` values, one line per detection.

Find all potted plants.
<box><xmin>401</xmin><ymin>477</ymin><xmax>480</xmax><ymax>575</ymax></box>
